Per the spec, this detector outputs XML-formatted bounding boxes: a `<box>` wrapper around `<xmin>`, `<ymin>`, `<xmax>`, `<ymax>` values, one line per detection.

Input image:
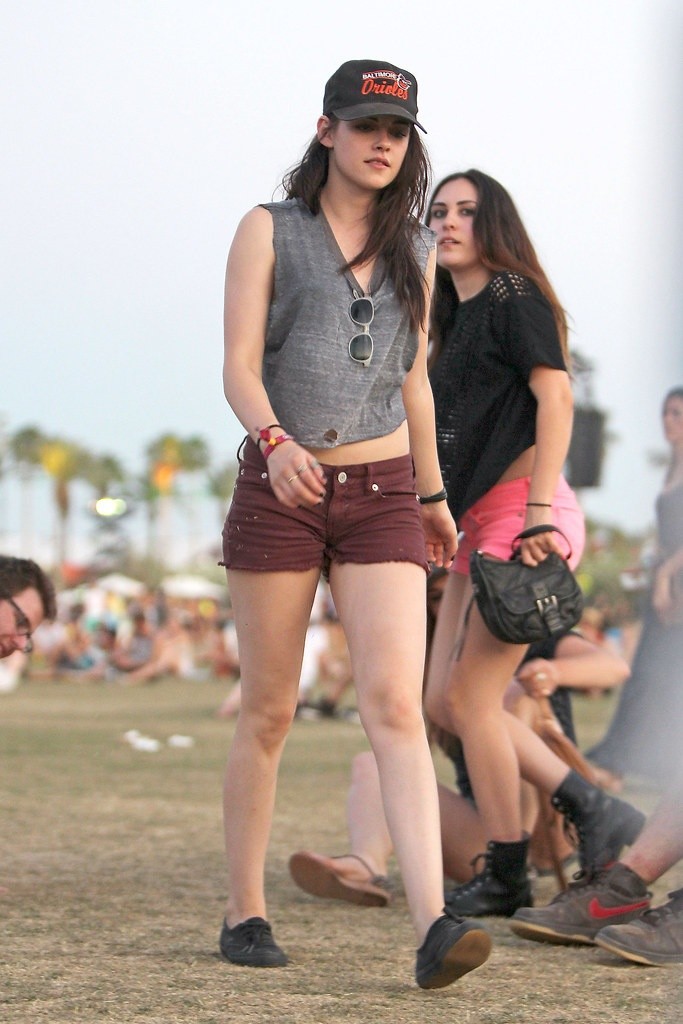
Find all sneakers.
<box><xmin>595</xmin><ymin>885</ymin><xmax>683</xmax><ymax>965</ymax></box>
<box><xmin>415</xmin><ymin>906</ymin><xmax>491</xmax><ymax>989</ymax></box>
<box><xmin>512</xmin><ymin>867</ymin><xmax>652</xmax><ymax>946</ymax></box>
<box><xmin>219</xmin><ymin>915</ymin><xmax>286</xmax><ymax>966</ymax></box>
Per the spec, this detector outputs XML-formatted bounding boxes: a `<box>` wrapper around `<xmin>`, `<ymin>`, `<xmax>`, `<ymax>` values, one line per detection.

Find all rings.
<box><xmin>297</xmin><ymin>464</ymin><xmax>308</xmax><ymax>474</ymax></box>
<box><xmin>287</xmin><ymin>475</ymin><xmax>298</xmax><ymax>483</ymax></box>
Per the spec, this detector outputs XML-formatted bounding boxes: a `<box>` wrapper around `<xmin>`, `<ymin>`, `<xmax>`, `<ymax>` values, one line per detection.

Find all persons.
<box><xmin>509</xmin><ymin>776</ymin><xmax>683</xmax><ymax>970</ymax></box>
<box><xmin>288</xmin><ymin>568</ymin><xmax>598</xmax><ymax>915</ymax></box>
<box><xmin>0</xmin><ymin>542</ymin><xmax>357</xmax><ymax>719</ymax></box>
<box><xmin>422</xmin><ymin>166</ymin><xmax>649</xmax><ymax>923</ymax></box>
<box><xmin>591</xmin><ymin>386</ymin><xmax>683</xmax><ymax>787</ymax></box>
<box><xmin>215</xmin><ymin>52</ymin><xmax>498</xmax><ymax>1000</ymax></box>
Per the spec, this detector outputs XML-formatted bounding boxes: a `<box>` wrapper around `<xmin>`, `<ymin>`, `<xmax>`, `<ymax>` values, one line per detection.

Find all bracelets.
<box><xmin>257</xmin><ymin>434</ymin><xmax>295</xmax><ymax>459</ymax></box>
<box><xmin>417</xmin><ymin>487</ymin><xmax>449</xmax><ymax>505</ymax></box>
<box><xmin>249</xmin><ymin>424</ymin><xmax>284</xmax><ymax>444</ymax></box>
<box><xmin>524</xmin><ymin>501</ymin><xmax>554</xmax><ymax>510</ymax></box>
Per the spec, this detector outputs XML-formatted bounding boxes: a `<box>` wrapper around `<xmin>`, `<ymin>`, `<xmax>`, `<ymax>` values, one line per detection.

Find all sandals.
<box><xmin>287</xmin><ymin>851</ymin><xmax>391</xmax><ymax>905</ymax></box>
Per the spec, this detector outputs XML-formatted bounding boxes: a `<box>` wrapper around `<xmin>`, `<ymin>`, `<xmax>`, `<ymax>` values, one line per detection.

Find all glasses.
<box><xmin>349</xmin><ymin>289</ymin><xmax>375</xmax><ymax>367</ymax></box>
<box><xmin>7</xmin><ymin>597</ymin><xmax>34</xmax><ymax>653</ymax></box>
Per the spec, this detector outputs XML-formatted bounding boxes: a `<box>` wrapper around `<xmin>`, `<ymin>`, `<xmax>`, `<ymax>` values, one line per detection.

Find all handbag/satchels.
<box><xmin>469</xmin><ymin>525</ymin><xmax>583</xmax><ymax>645</ymax></box>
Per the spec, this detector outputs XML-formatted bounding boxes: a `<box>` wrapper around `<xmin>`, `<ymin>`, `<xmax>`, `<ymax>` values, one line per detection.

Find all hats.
<box><xmin>323</xmin><ymin>59</ymin><xmax>427</xmax><ymax>134</ymax></box>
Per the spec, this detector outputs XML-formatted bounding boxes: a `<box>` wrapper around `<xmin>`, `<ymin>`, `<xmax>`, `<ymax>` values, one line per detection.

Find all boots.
<box><xmin>446</xmin><ymin>830</ymin><xmax>533</xmax><ymax>918</ymax></box>
<box><xmin>550</xmin><ymin>766</ymin><xmax>647</xmax><ymax>887</ymax></box>
